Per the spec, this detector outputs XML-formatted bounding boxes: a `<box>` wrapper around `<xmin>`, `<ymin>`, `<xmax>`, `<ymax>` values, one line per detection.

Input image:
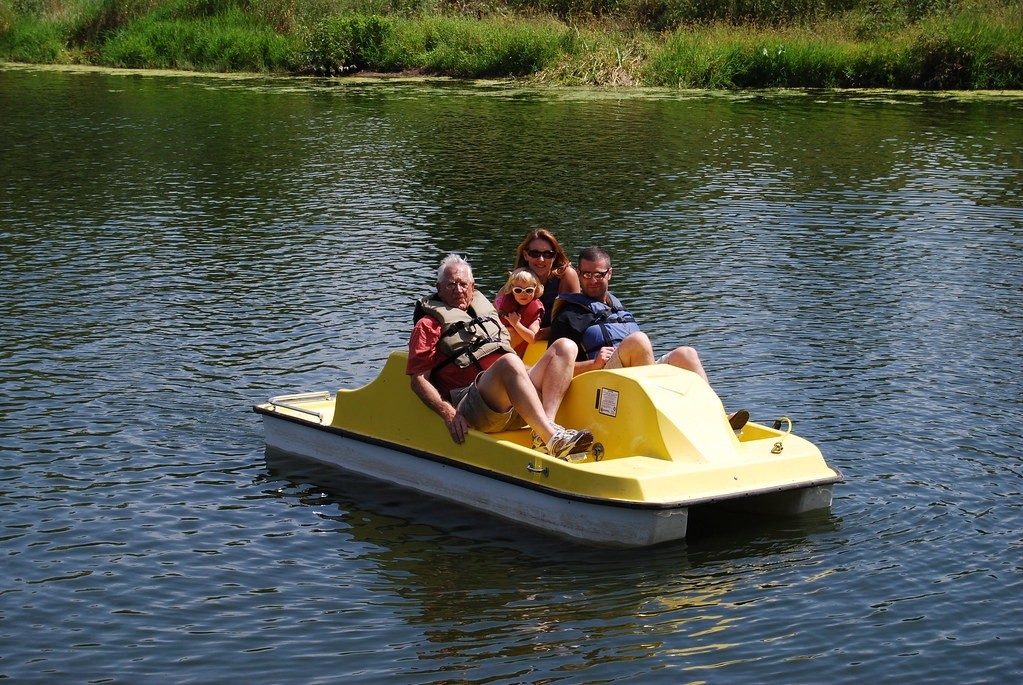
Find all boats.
<box><xmin>252</xmin><ymin>350</ymin><xmax>844</xmax><ymax>550</ymax></box>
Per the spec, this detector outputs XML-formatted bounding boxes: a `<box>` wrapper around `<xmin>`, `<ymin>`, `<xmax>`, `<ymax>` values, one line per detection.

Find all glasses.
<box><xmin>445</xmin><ymin>280</ymin><xmax>472</xmax><ymax>290</ymax></box>
<box><xmin>511</xmin><ymin>285</ymin><xmax>537</xmax><ymax>295</ymax></box>
<box><xmin>525</xmin><ymin>247</ymin><xmax>557</xmax><ymax>259</ymax></box>
<box><xmin>579</xmin><ymin>269</ymin><xmax>609</xmax><ymax>280</ymax></box>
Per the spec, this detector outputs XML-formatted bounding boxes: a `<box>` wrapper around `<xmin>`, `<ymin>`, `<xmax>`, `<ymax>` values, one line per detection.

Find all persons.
<box><xmin>495</xmin><ymin>228</ymin><xmax>581</xmax><ymax>340</ymax></box>
<box><xmin>497</xmin><ymin>267</ymin><xmax>544</xmax><ymax>358</ymax></box>
<box><xmin>546</xmin><ymin>247</ymin><xmax>749</xmax><ymax>429</ymax></box>
<box><xmin>405</xmin><ymin>254</ymin><xmax>593</xmax><ymax>460</ymax></box>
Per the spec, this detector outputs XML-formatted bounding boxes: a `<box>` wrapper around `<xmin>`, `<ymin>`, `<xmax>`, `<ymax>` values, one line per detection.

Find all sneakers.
<box><xmin>529</xmin><ymin>418</ymin><xmax>578</xmax><ymax>454</ymax></box>
<box><xmin>550</xmin><ymin>429</ymin><xmax>594</xmax><ymax>460</ymax></box>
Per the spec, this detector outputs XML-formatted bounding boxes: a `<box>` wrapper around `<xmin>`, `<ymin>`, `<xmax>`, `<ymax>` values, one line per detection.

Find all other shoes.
<box><xmin>728</xmin><ymin>408</ymin><xmax>749</xmax><ymax>430</ymax></box>
